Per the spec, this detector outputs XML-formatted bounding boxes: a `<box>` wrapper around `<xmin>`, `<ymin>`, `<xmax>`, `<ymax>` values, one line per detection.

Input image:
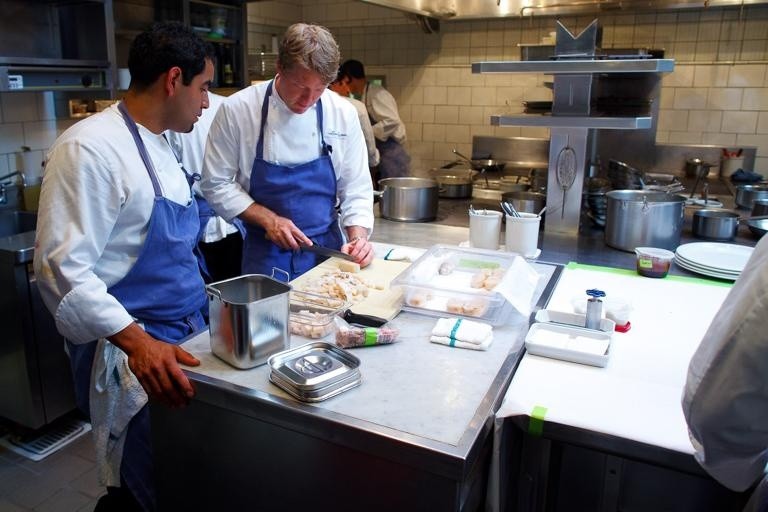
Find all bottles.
<box><xmin>223</xmin><ymin>64</ymin><xmax>233</xmax><ymax>84</ymax></box>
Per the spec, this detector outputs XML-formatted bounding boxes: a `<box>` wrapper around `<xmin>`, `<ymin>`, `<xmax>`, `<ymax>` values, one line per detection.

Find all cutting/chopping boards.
<box><xmin>289</xmin><ymin>241</ymin><xmax>429</xmax><ymax>329</ymax></box>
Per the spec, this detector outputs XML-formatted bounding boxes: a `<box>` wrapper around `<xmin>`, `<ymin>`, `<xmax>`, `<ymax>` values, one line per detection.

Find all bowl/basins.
<box><xmin>607</xmin><ymin>158</ymin><xmax>641</xmax><ymax>188</ymax></box>
<box><xmin>633</xmin><ymin>246</ymin><xmax>679</xmax><ymax>278</ymax></box>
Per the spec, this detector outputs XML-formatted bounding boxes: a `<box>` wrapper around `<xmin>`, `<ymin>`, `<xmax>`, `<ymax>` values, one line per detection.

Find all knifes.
<box><xmin>288</xmin><ymin>304</ymin><xmax>392</xmax><ymax>327</ymax></box>
<box><xmin>264</xmin><ymin>233</ymin><xmax>357</xmax><ymax>262</ymax></box>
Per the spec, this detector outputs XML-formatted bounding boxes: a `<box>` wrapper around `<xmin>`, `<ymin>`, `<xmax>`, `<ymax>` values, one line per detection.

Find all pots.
<box><xmin>691</xmin><ymin>209</ymin><xmax>741</xmax><ymax>241</ymax></box>
<box><xmin>368</xmin><ymin>175</ymin><xmax>446</xmax><ymax>222</ymax></box>
<box><xmin>451</xmin><ymin>148</ymin><xmax>506</xmax><ymax>172</ymax></box>
<box><xmin>425</xmin><ymin>167</ymin><xmax>480</xmax><ymax>198</ymax></box>
<box><xmin>500</xmin><ymin>191</ymin><xmax>546</xmax><ymax>228</ymax></box>
<box><xmin>734</xmin><ymin>185</ymin><xmax>768</xmax><ymax>237</ymax></box>
<box><xmin>684</xmin><ymin>151</ymin><xmax>718</xmax><ymax>178</ymax></box>
<box><xmin>719</xmin><ymin>156</ymin><xmax>753</xmax><ymax>177</ymax></box>
<box><xmin>586</xmin><ymin>189</ymin><xmax>688</xmax><ymax>250</ymax></box>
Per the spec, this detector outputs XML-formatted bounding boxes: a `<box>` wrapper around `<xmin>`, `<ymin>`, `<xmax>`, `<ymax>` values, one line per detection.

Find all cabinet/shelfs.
<box><xmin>70</xmin><ymin>0</ymin><xmax>248</xmax><ymax>100</ymax></box>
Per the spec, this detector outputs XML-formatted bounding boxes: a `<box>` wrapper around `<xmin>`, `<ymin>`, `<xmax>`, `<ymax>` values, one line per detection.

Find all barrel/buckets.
<box><xmin>205</xmin><ymin>267</ymin><xmax>292</xmax><ymax>370</ymax></box>
<box><xmin>721</xmin><ymin>154</ymin><xmax>743</xmax><ymax>177</ymax></box>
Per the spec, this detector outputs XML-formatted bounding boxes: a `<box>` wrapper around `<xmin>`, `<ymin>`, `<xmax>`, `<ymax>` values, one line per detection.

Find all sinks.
<box><xmin>473</xmin><ymin>187</ymin><xmax>504</xmax><ymax>201</ymax></box>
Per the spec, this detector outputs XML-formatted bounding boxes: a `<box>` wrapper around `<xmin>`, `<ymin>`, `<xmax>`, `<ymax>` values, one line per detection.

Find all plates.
<box><xmin>673</xmin><ymin>241</ymin><xmax>758</xmax><ymax>284</ymax></box>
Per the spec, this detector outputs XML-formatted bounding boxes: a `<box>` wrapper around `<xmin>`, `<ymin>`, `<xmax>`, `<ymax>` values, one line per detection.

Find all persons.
<box><xmin>339</xmin><ymin>59</ymin><xmax>409</xmax><ymax>184</ymax></box>
<box><xmin>34</xmin><ymin>18</ymin><xmax>218</xmax><ymax>488</ymax></box>
<box><xmin>680</xmin><ymin>228</ymin><xmax>768</xmax><ymax>512</ymax></box>
<box><xmin>198</xmin><ymin>19</ymin><xmax>379</xmax><ymax>283</ymax></box>
<box><xmin>329</xmin><ymin>63</ymin><xmax>382</xmax><ymax>184</ymax></box>
<box><xmin>161</xmin><ymin>86</ymin><xmax>247</xmax><ymax>280</ymax></box>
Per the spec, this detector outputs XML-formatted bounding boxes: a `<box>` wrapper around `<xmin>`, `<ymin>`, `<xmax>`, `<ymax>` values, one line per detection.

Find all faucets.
<box><xmin>450</xmin><ymin>147</ymin><xmax>477</xmax><ymax>169</ymax></box>
